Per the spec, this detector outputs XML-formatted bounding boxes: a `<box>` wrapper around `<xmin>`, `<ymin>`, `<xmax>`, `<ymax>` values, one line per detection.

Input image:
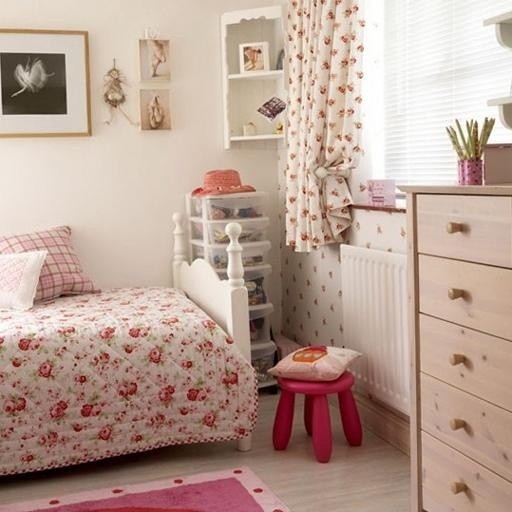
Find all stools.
<box><xmin>272</xmin><ymin>368</ymin><xmax>364</xmax><ymax>464</ymax></box>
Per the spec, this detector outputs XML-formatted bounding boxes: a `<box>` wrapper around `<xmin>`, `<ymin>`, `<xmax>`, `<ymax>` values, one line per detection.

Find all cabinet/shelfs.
<box><xmin>398</xmin><ymin>184</ymin><xmax>511</xmax><ymax>512</ymax></box>
<box><xmin>218</xmin><ymin>2</ymin><xmax>287</xmax><ymax>149</ymax></box>
<box><xmin>484</xmin><ymin>8</ymin><xmax>512</xmax><ymax>128</ymax></box>
<box><xmin>184</xmin><ymin>188</ymin><xmax>279</xmax><ymax>396</ymax></box>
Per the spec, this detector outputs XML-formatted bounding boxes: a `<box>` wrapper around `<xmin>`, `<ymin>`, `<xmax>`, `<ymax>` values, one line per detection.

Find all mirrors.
<box><xmin>0</xmin><ymin>225</ymin><xmax>101</xmax><ymax>305</ymax></box>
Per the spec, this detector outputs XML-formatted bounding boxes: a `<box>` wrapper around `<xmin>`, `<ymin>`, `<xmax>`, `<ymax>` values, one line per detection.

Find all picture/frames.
<box><xmin>239</xmin><ymin>40</ymin><xmax>272</xmax><ymax>74</ymax></box>
<box><xmin>1</xmin><ymin>25</ymin><xmax>94</xmax><ymax>139</ymax></box>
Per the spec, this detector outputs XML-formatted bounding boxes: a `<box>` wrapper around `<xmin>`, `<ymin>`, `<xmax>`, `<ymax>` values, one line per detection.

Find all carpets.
<box><xmin>1</xmin><ymin>462</ymin><xmax>294</xmax><ymax>512</ymax></box>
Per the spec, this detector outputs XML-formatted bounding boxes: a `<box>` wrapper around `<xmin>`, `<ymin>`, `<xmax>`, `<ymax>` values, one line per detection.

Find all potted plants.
<box><xmin>444</xmin><ymin>115</ymin><xmax>498</xmax><ymax>187</ymax></box>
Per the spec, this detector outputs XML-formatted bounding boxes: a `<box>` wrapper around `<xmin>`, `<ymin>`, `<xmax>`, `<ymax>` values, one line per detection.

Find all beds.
<box><xmin>0</xmin><ymin>211</ymin><xmax>261</xmax><ymax>479</ymax></box>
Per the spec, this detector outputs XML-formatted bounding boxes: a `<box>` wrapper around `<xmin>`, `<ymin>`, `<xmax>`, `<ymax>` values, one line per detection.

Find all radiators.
<box><xmin>338</xmin><ymin>241</ymin><xmax>410</xmax><ymax>419</ymax></box>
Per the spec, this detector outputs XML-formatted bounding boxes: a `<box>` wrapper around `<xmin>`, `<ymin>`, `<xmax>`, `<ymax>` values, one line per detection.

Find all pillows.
<box><xmin>0</xmin><ymin>248</ymin><xmax>49</xmax><ymax>314</ymax></box>
<box><xmin>267</xmin><ymin>343</ymin><xmax>364</xmax><ymax>382</ymax></box>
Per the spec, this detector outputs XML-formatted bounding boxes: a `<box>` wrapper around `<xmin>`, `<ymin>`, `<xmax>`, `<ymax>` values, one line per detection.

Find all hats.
<box><xmin>191</xmin><ymin>169</ymin><xmax>256</xmax><ymax>197</ymax></box>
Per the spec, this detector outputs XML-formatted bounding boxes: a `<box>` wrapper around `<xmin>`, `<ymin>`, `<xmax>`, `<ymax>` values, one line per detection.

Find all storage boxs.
<box><xmin>363</xmin><ymin>178</ymin><xmax>397</xmax><ymax>207</ymax></box>
